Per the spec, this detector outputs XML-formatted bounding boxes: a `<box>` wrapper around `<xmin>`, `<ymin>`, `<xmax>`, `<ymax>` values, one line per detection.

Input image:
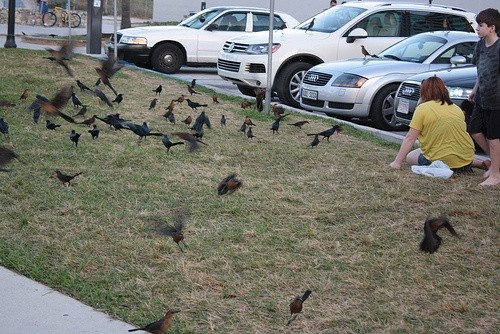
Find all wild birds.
<box><xmin>307</xmin><ymin>18</ymin><xmax>316</xmax><ymax>30</ymax></box>
<box><xmin>360</xmin><ymin>44</ymin><xmax>371</xmax><ymax>58</ymax></box>
<box><xmin>127</xmin><ymin>309</ymin><xmax>181</xmax><ymax>334</ymax></box>
<box><xmin>0</xmin><ymin>30</ymin><xmax>345</xmax><ymax>173</ymax></box>
<box><xmin>442</xmin><ymin>18</ymin><xmax>448</xmax><ymax>31</ymax></box>
<box><xmin>289</xmin><ymin>289</ymin><xmax>312</xmax><ymax>319</ymax></box>
<box><xmin>418</xmin><ymin>215</ymin><xmax>460</xmax><ymax>255</ymax></box>
<box><xmin>157</xmin><ymin>216</ymin><xmax>188</xmax><ymax>253</ymax></box>
<box><xmin>217</xmin><ymin>173</ymin><xmax>244</xmax><ymax>196</ymax></box>
<box><xmin>54</xmin><ymin>168</ymin><xmax>84</xmax><ymax>188</ymax></box>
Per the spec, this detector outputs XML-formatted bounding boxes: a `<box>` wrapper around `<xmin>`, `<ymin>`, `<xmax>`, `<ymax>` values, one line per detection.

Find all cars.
<box><xmin>393</xmin><ymin>63</ymin><xmax>478</xmax><ymax>128</ymax></box>
<box><xmin>216</xmin><ymin>0</ymin><xmax>483</xmax><ymax>112</ymax></box>
<box><xmin>106</xmin><ymin>5</ymin><xmax>303</xmax><ymax>74</ymax></box>
<box><xmin>298</xmin><ymin>29</ymin><xmax>486</xmax><ymax>129</ymax></box>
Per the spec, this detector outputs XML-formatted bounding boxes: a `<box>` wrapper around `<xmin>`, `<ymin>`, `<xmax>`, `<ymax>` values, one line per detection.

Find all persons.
<box><xmin>389</xmin><ymin>76</ymin><xmax>492</xmax><ymax>174</ymax></box>
<box><xmin>32</xmin><ymin>0</ymin><xmax>54</xmax><ymax>26</ymax></box>
<box><xmin>467</xmin><ymin>7</ymin><xmax>500</xmax><ymax>186</ymax></box>
<box><xmin>330</xmin><ymin>0</ymin><xmax>337</xmax><ymax>7</ymax></box>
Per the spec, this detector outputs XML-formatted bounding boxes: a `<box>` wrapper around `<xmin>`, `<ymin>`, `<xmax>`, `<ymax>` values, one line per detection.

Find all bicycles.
<box><xmin>42</xmin><ymin>6</ymin><xmax>82</xmax><ymax>28</ymax></box>
